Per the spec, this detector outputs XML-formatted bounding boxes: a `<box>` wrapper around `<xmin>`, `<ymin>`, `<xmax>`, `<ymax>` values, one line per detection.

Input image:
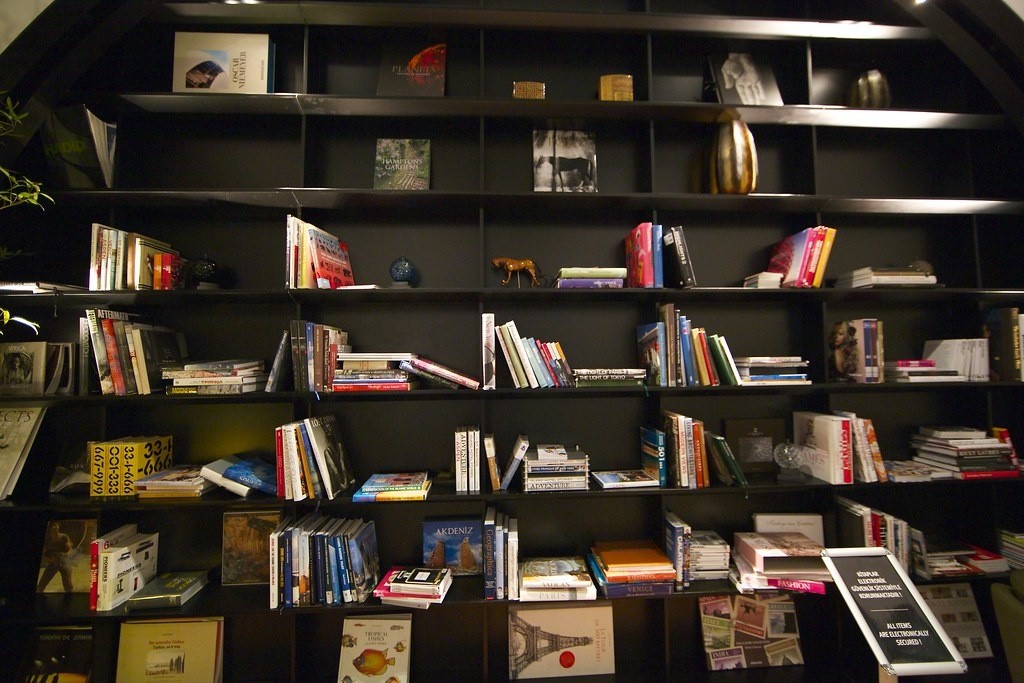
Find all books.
<box><xmin>0</xmin><ymin>33</ymin><xmax>1024</xmax><ymax>683</ymax></box>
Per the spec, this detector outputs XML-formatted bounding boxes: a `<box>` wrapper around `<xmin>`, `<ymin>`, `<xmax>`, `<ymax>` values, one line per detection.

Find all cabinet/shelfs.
<box><xmin>0</xmin><ymin>0</ymin><xmax>1024</xmax><ymax>683</ymax></box>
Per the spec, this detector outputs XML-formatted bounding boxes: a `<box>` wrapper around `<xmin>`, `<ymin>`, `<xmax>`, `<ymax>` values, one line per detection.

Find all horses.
<box><xmin>536</xmin><ymin>154</ymin><xmax>595</xmax><ymax>190</ymax></box>
<box><xmin>491</xmin><ymin>256</ymin><xmax>543</xmax><ymax>288</ymax></box>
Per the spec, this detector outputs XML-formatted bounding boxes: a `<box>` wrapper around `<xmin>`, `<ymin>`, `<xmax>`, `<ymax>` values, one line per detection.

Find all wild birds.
<box><xmin>427</xmin><ymin>538</ymin><xmax>478</xmax><ymax>570</ymax></box>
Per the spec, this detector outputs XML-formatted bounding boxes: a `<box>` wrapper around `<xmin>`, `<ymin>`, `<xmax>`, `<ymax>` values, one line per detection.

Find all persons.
<box><xmin>37</xmin><ymin>522</ymin><xmax>74</xmax><ymax>592</ymax></box>
<box><xmin>3</xmin><ymin>353</ymin><xmax>33</xmax><ymax>383</ymax></box>
<box><xmin>721</xmin><ymin>52</ymin><xmax>765</xmax><ymax>105</ymax></box>
<box><xmin>825</xmin><ymin>321</ymin><xmax>859</xmax><ymax>382</ymax></box>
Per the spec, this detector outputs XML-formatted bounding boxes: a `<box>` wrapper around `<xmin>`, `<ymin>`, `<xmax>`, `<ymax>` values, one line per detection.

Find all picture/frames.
<box><xmin>0</xmin><ymin>341</ymin><xmax>47</xmax><ymax>394</ymax></box>
